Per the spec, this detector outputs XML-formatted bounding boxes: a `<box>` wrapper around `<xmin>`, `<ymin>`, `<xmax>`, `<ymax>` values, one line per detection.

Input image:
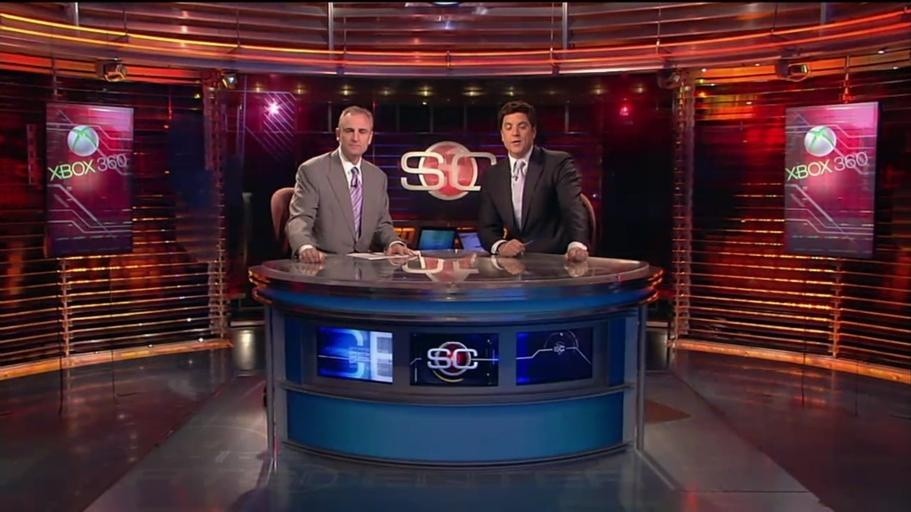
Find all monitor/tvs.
<box><xmin>417</xmin><ymin>227</ymin><xmax>456</xmax><ymax>251</ymax></box>
<box><xmin>456</xmin><ymin>229</ymin><xmax>486</xmax><ymax>253</ymax></box>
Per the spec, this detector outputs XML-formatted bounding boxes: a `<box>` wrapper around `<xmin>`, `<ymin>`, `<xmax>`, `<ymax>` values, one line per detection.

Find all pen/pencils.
<box><xmin>522</xmin><ymin>240</ymin><xmax>534</xmax><ymax>247</ymax></box>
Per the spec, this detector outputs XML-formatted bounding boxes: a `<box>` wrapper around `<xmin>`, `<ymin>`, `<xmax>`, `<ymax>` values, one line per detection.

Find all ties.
<box><xmin>348</xmin><ymin>165</ymin><xmax>363</xmax><ymax>242</ymax></box>
<box><xmin>513</xmin><ymin>159</ymin><xmax>527</xmax><ymax>224</ymax></box>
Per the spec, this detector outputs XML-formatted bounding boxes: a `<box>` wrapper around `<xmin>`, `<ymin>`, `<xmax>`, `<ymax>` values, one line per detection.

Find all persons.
<box><xmin>285</xmin><ymin>255</ymin><xmax>402</xmax><ymax>284</ymax></box>
<box><xmin>473</xmin><ymin>100</ymin><xmax>589</xmax><ymax>259</ymax></box>
<box><xmin>284</xmin><ymin>106</ymin><xmax>417</xmax><ymax>265</ymax></box>
<box><xmin>479</xmin><ymin>250</ymin><xmax>590</xmax><ymax>280</ymax></box>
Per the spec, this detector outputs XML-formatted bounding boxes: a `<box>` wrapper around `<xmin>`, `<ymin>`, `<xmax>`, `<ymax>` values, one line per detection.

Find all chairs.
<box><xmin>270</xmin><ymin>188</ymin><xmax>298</xmax><ymax>259</ymax></box>
<box><xmin>579</xmin><ymin>192</ymin><xmax>598</xmax><ymax>257</ymax></box>
